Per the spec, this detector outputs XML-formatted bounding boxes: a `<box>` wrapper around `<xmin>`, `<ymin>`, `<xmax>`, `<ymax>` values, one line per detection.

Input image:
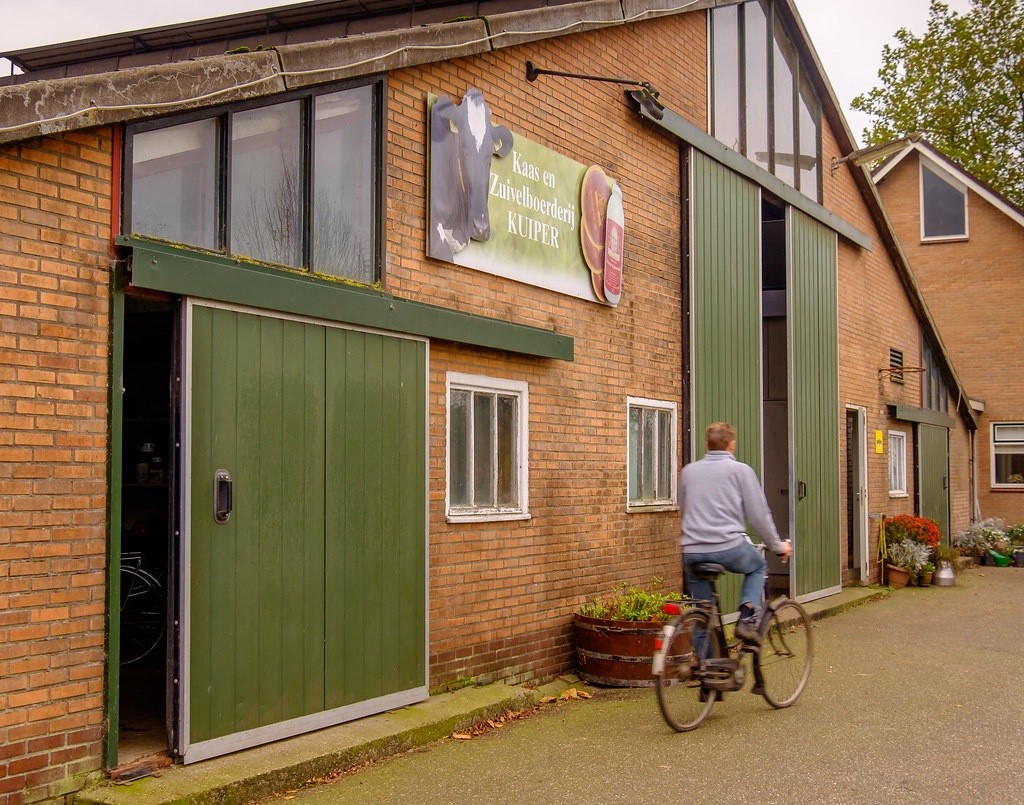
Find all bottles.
<box><xmin>604</xmin><ymin>183</ymin><xmax>624</xmax><ymax>304</ymax></box>
<box><xmin>781</xmin><ymin>538</ymin><xmax>791</xmax><ymax>563</ymax></box>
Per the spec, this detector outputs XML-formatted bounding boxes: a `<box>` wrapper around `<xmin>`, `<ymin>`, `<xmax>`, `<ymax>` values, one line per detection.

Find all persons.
<box><xmin>676</xmin><ymin>421</ymin><xmax>794</xmax><ymax>673</ymax></box>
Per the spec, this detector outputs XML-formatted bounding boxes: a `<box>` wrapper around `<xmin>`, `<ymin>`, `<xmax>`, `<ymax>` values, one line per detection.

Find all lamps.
<box><xmin>525</xmin><ymin>59</ymin><xmax>665</xmax><ymax>121</ymax></box>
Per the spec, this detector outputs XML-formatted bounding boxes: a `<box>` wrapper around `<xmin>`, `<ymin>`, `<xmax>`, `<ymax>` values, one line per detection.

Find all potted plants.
<box><xmin>571</xmin><ymin>573</ymin><xmax>696</xmax><ymax>690</ymax></box>
<box><xmin>920</xmin><ymin>561</ymin><xmax>936</xmax><ymax>587</ymax></box>
<box><xmin>956</xmin><ymin>516</ymin><xmax>1024</xmax><ymax>568</ymax></box>
<box><xmin>934</xmin><ymin>545</ymin><xmax>961</xmax><ymax>587</ymax></box>
<box><xmin>885</xmin><ymin>540</ymin><xmax>933</xmax><ymax>588</ymax></box>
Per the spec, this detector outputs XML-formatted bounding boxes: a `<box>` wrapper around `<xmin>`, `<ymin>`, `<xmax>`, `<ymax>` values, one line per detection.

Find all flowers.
<box><xmin>883</xmin><ymin>514</ymin><xmax>942</xmax><ymax>547</ymax></box>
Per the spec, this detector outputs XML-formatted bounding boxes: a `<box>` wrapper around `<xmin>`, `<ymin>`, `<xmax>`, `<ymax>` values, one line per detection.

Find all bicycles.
<box><xmin>652</xmin><ymin>531</ymin><xmax>816</xmax><ymax>732</ymax></box>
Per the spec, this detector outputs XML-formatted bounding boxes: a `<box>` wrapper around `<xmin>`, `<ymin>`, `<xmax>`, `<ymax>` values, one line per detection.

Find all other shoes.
<box><xmin>734</xmin><ymin>623</ymin><xmax>762</xmax><ymax>647</ymax></box>
